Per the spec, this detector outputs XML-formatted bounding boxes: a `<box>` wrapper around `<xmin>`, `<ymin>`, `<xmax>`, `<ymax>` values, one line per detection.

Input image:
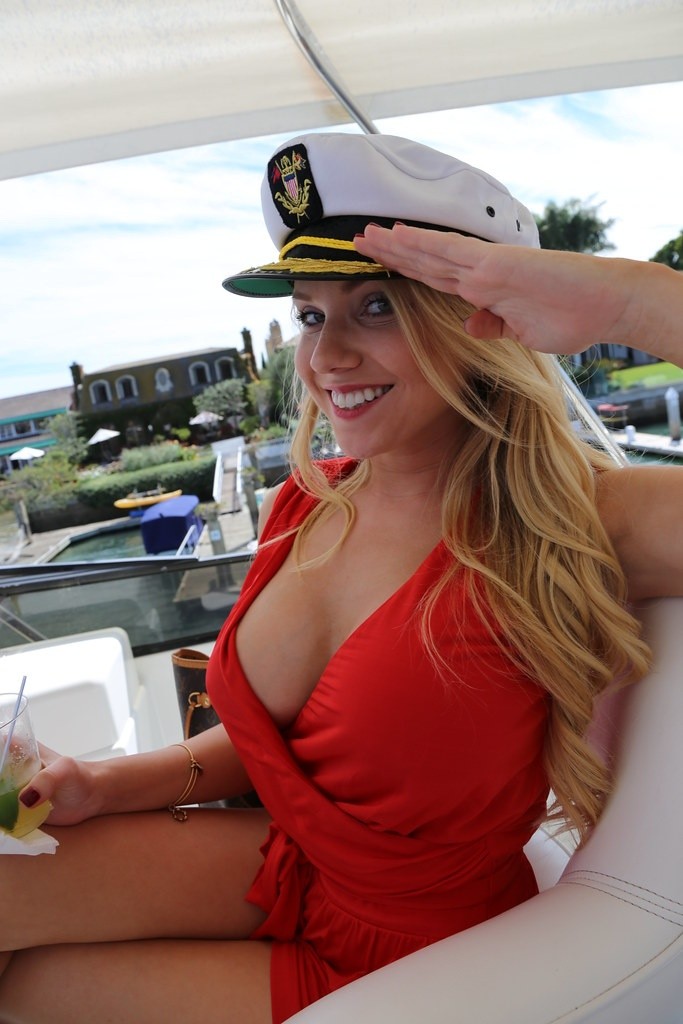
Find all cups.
<box><xmin>0</xmin><ymin>693</ymin><xmax>53</xmax><ymax>840</ymax></box>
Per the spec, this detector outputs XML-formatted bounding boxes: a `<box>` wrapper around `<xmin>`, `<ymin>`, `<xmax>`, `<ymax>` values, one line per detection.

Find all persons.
<box><xmin>0</xmin><ymin>131</ymin><xmax>683</xmax><ymax>1023</ymax></box>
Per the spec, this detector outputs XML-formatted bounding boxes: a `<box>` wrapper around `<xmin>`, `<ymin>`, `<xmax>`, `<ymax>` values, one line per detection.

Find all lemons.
<box><xmin>0</xmin><ymin>774</ymin><xmax>19</xmax><ymax>831</ymax></box>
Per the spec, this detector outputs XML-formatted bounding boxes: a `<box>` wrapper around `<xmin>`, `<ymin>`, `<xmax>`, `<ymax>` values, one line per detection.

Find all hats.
<box><xmin>223</xmin><ymin>132</ymin><xmax>542</xmax><ymax>297</ymax></box>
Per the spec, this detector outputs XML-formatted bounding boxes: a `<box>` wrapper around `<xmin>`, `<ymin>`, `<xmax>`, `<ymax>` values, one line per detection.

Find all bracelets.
<box><xmin>170</xmin><ymin>744</ymin><xmax>204</xmax><ymax>823</ymax></box>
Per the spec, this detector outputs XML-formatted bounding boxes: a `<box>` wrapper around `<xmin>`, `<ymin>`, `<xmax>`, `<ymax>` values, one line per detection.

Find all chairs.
<box><xmin>284</xmin><ymin>589</ymin><xmax>683</xmax><ymax>1022</ymax></box>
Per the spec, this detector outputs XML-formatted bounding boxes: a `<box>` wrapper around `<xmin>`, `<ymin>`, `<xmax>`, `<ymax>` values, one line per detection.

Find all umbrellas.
<box><xmin>189</xmin><ymin>410</ymin><xmax>225</xmax><ymax>432</ymax></box>
<box><xmin>85</xmin><ymin>428</ymin><xmax>121</xmax><ymax>447</ymax></box>
<box><xmin>9</xmin><ymin>446</ymin><xmax>46</xmax><ymax>461</ymax></box>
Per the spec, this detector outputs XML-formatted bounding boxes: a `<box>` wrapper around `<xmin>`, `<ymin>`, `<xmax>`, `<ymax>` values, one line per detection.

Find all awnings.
<box><xmin>0</xmin><ymin>437</ymin><xmax>60</xmax><ymax>456</ymax></box>
